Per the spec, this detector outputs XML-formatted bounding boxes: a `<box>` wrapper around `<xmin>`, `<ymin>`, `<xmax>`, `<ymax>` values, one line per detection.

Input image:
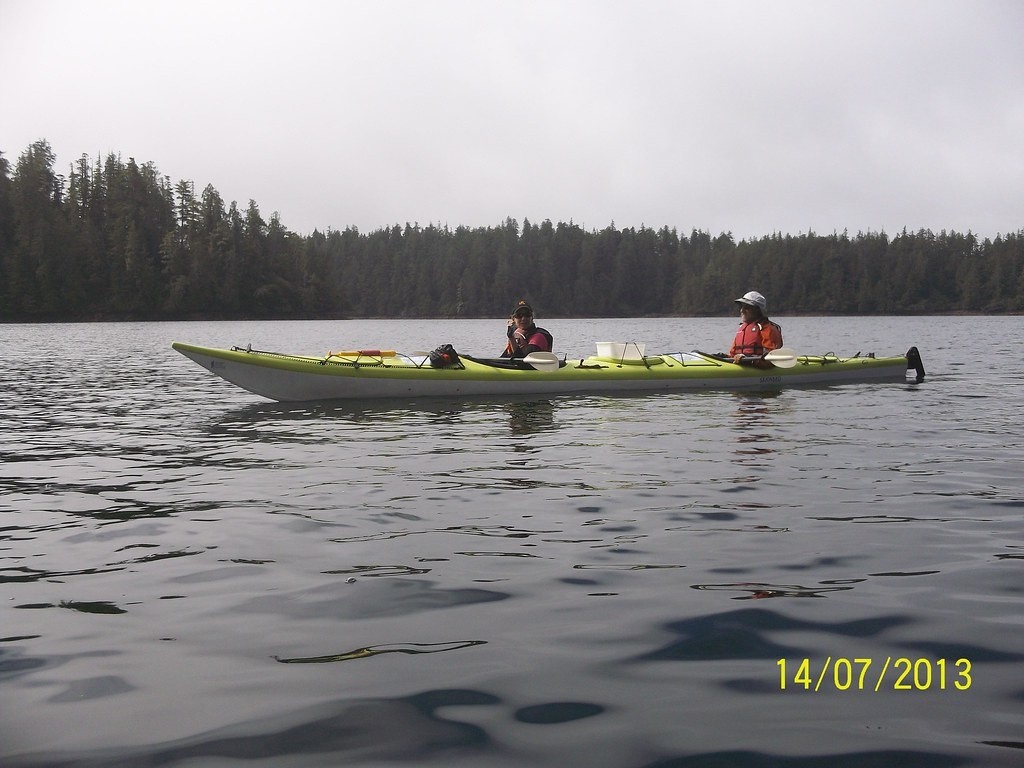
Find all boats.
<box><xmin>172</xmin><ymin>344</ymin><xmax>926</xmax><ymax>402</ymax></box>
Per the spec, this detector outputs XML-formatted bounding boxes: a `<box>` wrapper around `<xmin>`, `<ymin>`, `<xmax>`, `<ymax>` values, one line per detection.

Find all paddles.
<box><xmin>413</xmin><ymin>349</ymin><xmax>560</xmax><ymax>374</ymax></box>
<box><xmin>722</xmin><ymin>348</ymin><xmax>797</xmax><ymax>369</ymax></box>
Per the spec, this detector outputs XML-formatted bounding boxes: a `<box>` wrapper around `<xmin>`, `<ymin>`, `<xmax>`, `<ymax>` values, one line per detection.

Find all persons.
<box><xmin>499</xmin><ymin>299</ymin><xmax>550</xmax><ymax>370</ymax></box>
<box><xmin>713</xmin><ymin>290</ymin><xmax>783</xmax><ymax>371</ymax></box>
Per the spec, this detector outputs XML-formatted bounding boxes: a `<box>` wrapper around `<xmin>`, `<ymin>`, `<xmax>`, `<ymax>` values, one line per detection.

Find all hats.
<box><xmin>733</xmin><ymin>290</ymin><xmax>766</xmax><ymax>309</ymax></box>
<box><xmin>511</xmin><ymin>298</ymin><xmax>533</xmax><ymax>312</ymax></box>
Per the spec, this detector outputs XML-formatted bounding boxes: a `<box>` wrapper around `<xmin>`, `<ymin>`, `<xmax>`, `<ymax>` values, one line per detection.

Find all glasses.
<box><xmin>512</xmin><ymin>310</ymin><xmax>533</xmax><ymax>319</ymax></box>
<box><xmin>737</xmin><ymin>303</ymin><xmax>754</xmax><ymax>309</ymax></box>
